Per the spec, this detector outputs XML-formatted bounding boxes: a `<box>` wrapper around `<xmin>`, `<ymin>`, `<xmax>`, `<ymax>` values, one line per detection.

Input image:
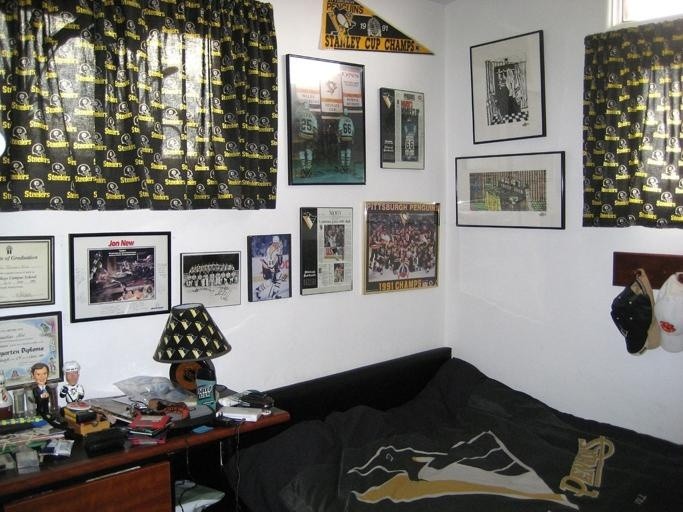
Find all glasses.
<box><xmin>154</xmin><ymin>302</ymin><xmax>231</xmax><ymax>392</ymax></box>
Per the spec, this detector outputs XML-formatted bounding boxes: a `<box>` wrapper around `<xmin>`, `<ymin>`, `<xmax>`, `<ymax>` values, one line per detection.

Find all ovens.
<box><xmin>178</xmin><ymin>345</ymin><xmax>683</xmax><ymax>511</ymax></box>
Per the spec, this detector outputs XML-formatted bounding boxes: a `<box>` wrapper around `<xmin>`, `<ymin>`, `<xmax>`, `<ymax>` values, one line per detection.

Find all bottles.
<box><xmin>610</xmin><ymin>269</ymin><xmax>661</xmax><ymax>354</ymax></box>
<box><xmin>655</xmin><ymin>271</ymin><xmax>683</xmax><ymax>353</ymax></box>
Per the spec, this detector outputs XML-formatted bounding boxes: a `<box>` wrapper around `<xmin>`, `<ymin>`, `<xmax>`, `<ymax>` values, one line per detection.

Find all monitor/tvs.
<box><xmin>215</xmin><ymin>406</ymin><xmax>262</xmax><ymax>422</ymax></box>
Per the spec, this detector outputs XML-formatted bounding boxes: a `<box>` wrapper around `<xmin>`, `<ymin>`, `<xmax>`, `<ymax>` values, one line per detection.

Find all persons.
<box><xmin>368</xmin><ymin>213</ymin><xmax>435</xmax><ymax>275</ymax></box>
<box><xmin>336</xmin><ymin>108</ymin><xmax>355</xmax><ymax>173</ymax></box>
<box><xmin>324</xmin><ymin>228</ymin><xmax>340</xmax><ymax>261</ymax></box>
<box><xmin>255</xmin><ymin>236</ymin><xmax>283</xmax><ymax>299</ymax></box>
<box><xmin>185</xmin><ymin>262</ymin><xmax>237</xmax><ymax>287</ymax></box>
<box><xmin>295</xmin><ymin>102</ymin><xmax>318</xmax><ymax>177</ymax></box>
<box><xmin>89</xmin><ymin>252</ymin><xmax>154</xmax><ymax>302</ymax></box>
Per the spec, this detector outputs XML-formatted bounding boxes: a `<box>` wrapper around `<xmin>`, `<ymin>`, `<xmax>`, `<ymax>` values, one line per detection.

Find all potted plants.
<box><xmin>246</xmin><ymin>232</ymin><xmax>292</xmax><ymax>300</ymax></box>
<box><xmin>284</xmin><ymin>53</ymin><xmax>367</xmax><ymax>186</ymax></box>
<box><xmin>454</xmin><ymin>148</ymin><xmax>565</xmax><ymax>231</ymax></box>
<box><xmin>0</xmin><ymin>311</ymin><xmax>64</xmax><ymax>391</ymax></box>
<box><xmin>68</xmin><ymin>230</ymin><xmax>173</xmax><ymax>324</ymax></box>
<box><xmin>468</xmin><ymin>29</ymin><xmax>547</xmax><ymax>146</ymax></box>
<box><xmin>178</xmin><ymin>250</ymin><xmax>242</xmax><ymax>310</ymax></box>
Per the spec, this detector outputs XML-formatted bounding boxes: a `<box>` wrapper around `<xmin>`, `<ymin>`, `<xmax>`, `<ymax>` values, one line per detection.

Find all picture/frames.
<box><xmin>1</xmin><ymin>384</ymin><xmax>291</xmax><ymax>512</ymax></box>
